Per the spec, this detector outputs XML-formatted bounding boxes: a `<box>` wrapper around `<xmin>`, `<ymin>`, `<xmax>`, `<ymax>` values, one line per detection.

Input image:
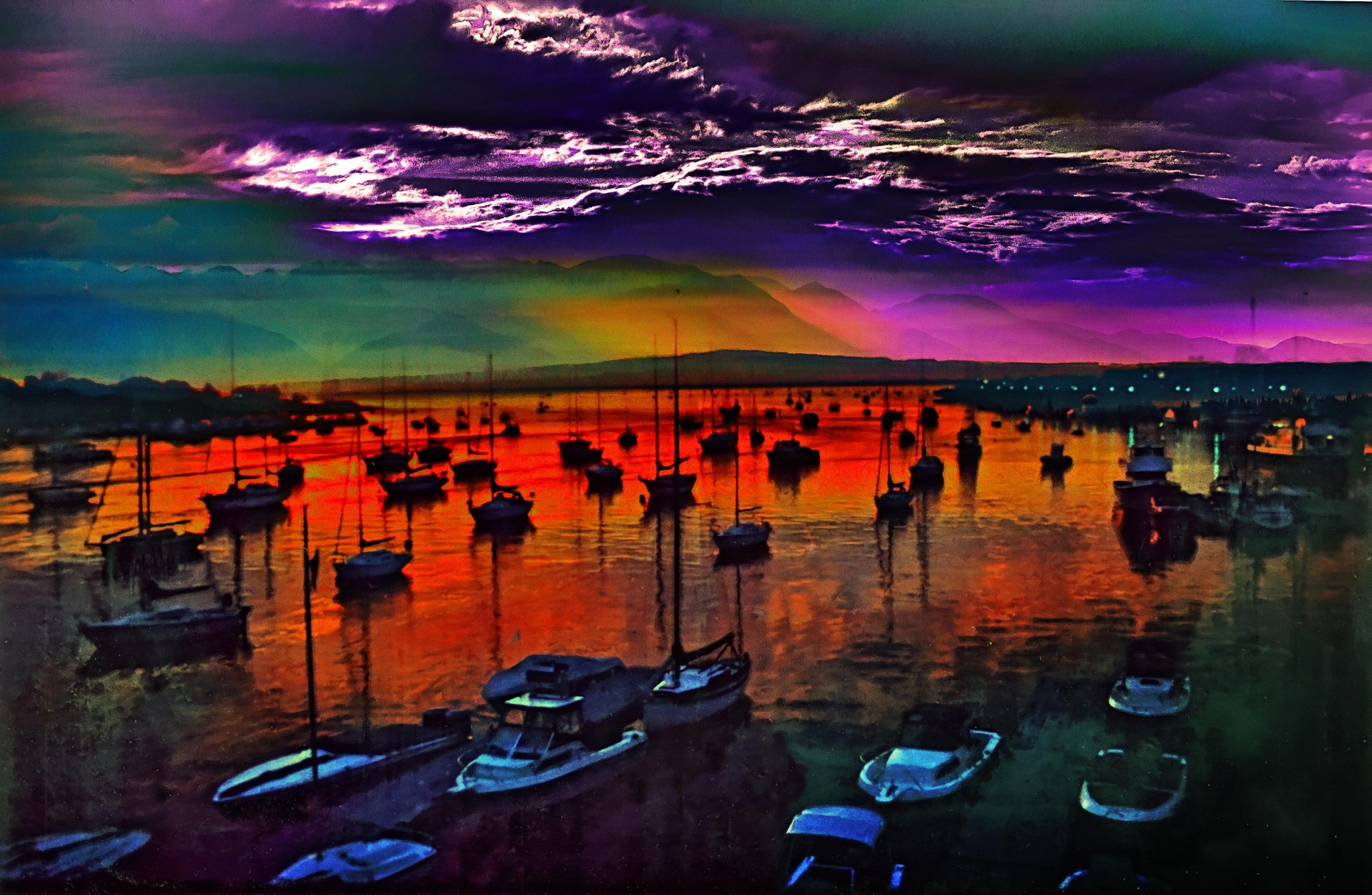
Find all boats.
<box><xmin>73</xmin><ymin>574</ymin><xmax>250</xmax><ymax>657</ymax></box>
<box><xmin>1030</xmin><ymin>442</ymin><xmax>1074</xmax><ymax>476</ymax></box>
<box><xmin>1106</xmin><ymin>615</ymin><xmax>1193</xmax><ymax>725</ymax></box>
<box><xmin>1055</xmin><ymin>864</ymin><xmax>1155</xmax><ymax>895</ymax></box>
<box><xmin>1074</xmin><ymin>744</ymin><xmax>1188</xmax><ymax>827</ymax></box>
<box><xmin>29</xmin><ymin>434</ymin><xmax>120</xmax><ymax>466</ymax></box>
<box><xmin>850</xmin><ymin>696</ymin><xmax>1006</xmax><ymax>806</ymax></box>
<box><xmin>1</xmin><ymin>817</ymin><xmax>158</xmax><ymax>895</ymax></box>
<box><xmin>775</xmin><ymin>802</ymin><xmax>906</xmax><ymax>895</ymax></box>
<box><xmin>29</xmin><ymin>462</ymin><xmax>96</xmax><ymax>513</ymax></box>
<box><xmin>1117</xmin><ymin>415</ymin><xmax>1358</xmax><ymax>549</ymax></box>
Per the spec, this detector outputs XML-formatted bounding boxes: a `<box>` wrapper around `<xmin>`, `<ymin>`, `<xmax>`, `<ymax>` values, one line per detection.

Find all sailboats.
<box><xmin>196</xmin><ymin>299</ymin><xmax>984</xmax><ymax>895</ymax></box>
